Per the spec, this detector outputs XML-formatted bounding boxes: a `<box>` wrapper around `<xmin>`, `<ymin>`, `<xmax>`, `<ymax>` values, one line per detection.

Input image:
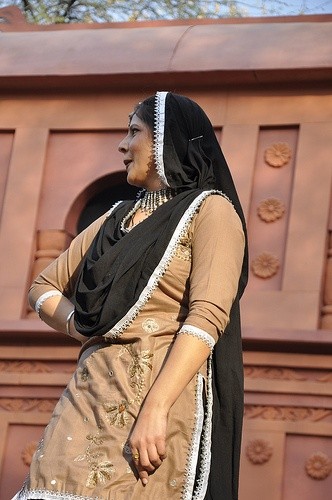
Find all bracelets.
<box><xmin>66</xmin><ymin>307</ymin><xmax>75</xmax><ymax>336</ymax></box>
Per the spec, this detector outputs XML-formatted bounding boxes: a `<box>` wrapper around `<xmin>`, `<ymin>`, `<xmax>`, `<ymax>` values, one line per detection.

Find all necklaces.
<box><xmin>139</xmin><ymin>187</ymin><xmax>187</xmax><ymax>217</ymax></box>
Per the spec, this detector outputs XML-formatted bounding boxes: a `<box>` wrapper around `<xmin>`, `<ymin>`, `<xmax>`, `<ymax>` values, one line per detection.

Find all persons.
<box><xmin>12</xmin><ymin>87</ymin><xmax>250</xmax><ymax>500</ymax></box>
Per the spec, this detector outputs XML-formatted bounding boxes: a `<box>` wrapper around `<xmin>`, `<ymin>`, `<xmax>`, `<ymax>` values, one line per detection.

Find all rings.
<box><xmin>131</xmin><ymin>452</ymin><xmax>140</xmax><ymax>460</ymax></box>
